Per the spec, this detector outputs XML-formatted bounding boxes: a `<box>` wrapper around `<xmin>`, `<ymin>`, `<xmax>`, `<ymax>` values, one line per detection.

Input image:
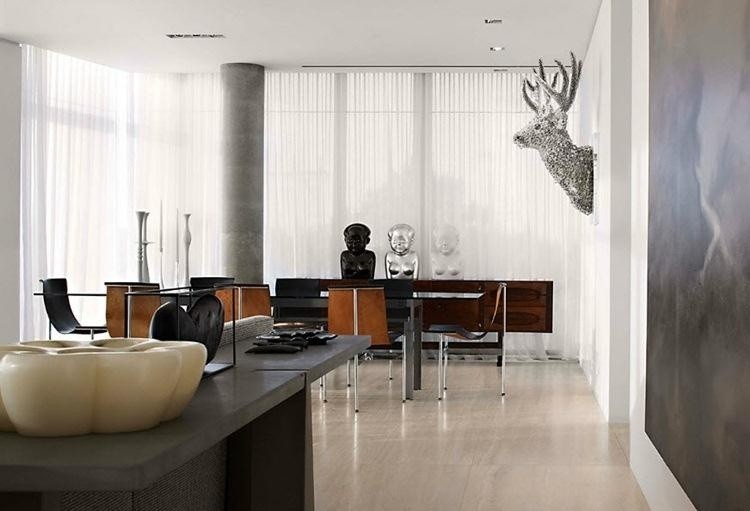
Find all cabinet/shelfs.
<box><xmin>420</xmin><ymin>280</ymin><xmax>553</xmax><ymax>333</ymax></box>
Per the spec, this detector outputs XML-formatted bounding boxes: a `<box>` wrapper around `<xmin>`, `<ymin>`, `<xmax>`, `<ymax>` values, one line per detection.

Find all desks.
<box><xmin>34</xmin><ymin>284</ymin><xmax>489</xmax><ymax>390</ymax></box>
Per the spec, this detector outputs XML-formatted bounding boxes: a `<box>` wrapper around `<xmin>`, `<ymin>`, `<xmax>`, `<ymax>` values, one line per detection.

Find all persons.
<box><xmin>383</xmin><ymin>223</ymin><xmax>422</xmax><ymax>281</ymax></box>
<box><xmin>339</xmin><ymin>223</ymin><xmax>376</xmax><ymax>280</ymax></box>
<box><xmin>429</xmin><ymin>224</ymin><xmax>466</xmax><ymax>282</ymax></box>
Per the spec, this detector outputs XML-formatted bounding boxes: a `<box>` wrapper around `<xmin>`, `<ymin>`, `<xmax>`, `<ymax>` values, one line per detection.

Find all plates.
<box><xmin>254</xmin><ymin>329</ymin><xmax>339</xmax><ymax>347</ymax></box>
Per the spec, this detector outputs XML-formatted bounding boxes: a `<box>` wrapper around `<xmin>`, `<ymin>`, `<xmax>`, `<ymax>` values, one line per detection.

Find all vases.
<box><xmin>134</xmin><ymin>211</ymin><xmax>154</xmax><ymax>284</ymax></box>
<box><xmin>181</xmin><ymin>214</ymin><xmax>193</xmax><ymax>285</ymax></box>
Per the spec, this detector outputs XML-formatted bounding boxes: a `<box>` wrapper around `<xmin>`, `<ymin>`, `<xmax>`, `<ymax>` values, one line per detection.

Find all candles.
<box><xmin>159</xmin><ymin>200</ymin><xmax>163</xmax><ymax>248</ymax></box>
<box><xmin>175</xmin><ymin>208</ymin><xmax>180</xmax><ymax>257</ymax></box>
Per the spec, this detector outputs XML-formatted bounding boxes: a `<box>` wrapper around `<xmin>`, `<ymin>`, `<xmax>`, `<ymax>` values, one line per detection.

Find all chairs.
<box><xmin>427</xmin><ymin>283</ymin><xmax>509</xmax><ymax>402</ymax></box>
<box><xmin>322</xmin><ymin>285</ymin><xmax>406</xmax><ymax>412</ymax></box>
<box><xmin>190</xmin><ymin>277</ymin><xmax>235</xmax><ymax>308</ymax></box>
<box><xmin>39</xmin><ymin>278</ymin><xmax>109</xmax><ymax>341</ymax></box>
<box><xmin>273</xmin><ymin>279</ymin><xmax>322</xmax><ymax>322</ymax></box>
<box><xmin>344</xmin><ymin>280</ymin><xmax>413</xmax><ymax>388</ymax></box>
<box><xmin>214</xmin><ymin>282</ymin><xmax>272</xmax><ymax>324</ymax></box>
<box><xmin>104</xmin><ymin>282</ymin><xmax>162</xmax><ymax>338</ymax></box>
